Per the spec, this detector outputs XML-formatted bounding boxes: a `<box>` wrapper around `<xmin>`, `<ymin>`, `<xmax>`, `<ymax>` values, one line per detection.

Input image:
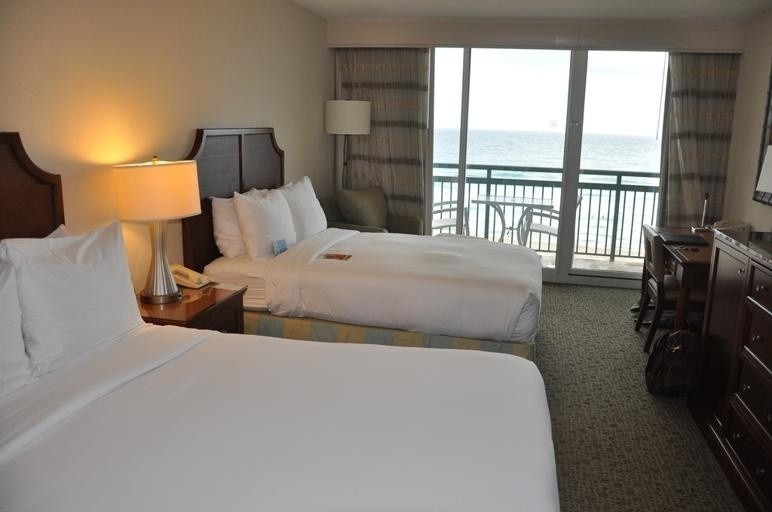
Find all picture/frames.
<box><xmin>752</xmin><ymin>62</ymin><xmax>772</xmax><ymax>205</ymax></box>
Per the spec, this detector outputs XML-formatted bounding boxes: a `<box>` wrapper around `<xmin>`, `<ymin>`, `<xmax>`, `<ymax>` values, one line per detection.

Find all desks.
<box><xmin>631</xmin><ymin>225</ymin><xmax>714</xmax><ymax>332</ymax></box>
<box><xmin>471</xmin><ymin>197</ymin><xmax>554</xmax><ymax>247</ymax></box>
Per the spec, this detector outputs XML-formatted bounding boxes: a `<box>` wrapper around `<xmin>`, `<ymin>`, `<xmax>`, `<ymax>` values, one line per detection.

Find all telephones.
<box><xmin>170</xmin><ymin>264</ymin><xmax>213</xmax><ymax>289</ymax></box>
<box><xmin>714</xmin><ymin>218</ymin><xmax>744</xmax><ymax>228</ymax></box>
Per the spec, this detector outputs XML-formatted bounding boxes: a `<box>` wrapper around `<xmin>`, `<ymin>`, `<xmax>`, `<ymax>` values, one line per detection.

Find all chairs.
<box><xmin>635</xmin><ymin>223</ymin><xmax>708</xmax><ymax>352</ymax></box>
<box><xmin>432</xmin><ymin>201</ymin><xmax>469</xmax><ymax>236</ymax></box>
<box><xmin>319</xmin><ymin>195</ymin><xmax>425</xmax><ymax>235</ymax></box>
<box><xmin>521</xmin><ymin>194</ymin><xmax>583</xmax><ymax>249</ymax></box>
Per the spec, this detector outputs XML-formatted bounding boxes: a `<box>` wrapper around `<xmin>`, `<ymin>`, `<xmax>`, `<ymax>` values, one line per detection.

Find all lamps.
<box><xmin>755</xmin><ymin>145</ymin><xmax>772</xmax><ymax>193</ymax></box>
<box><xmin>112</xmin><ymin>155</ymin><xmax>202</xmax><ymax>305</ymax></box>
<box><xmin>324</xmin><ymin>100</ymin><xmax>372</xmax><ymax>194</ymax></box>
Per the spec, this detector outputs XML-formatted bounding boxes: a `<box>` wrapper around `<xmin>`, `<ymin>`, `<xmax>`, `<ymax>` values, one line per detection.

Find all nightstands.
<box><xmin>133</xmin><ymin>279</ymin><xmax>248</xmax><ymax>336</ymax></box>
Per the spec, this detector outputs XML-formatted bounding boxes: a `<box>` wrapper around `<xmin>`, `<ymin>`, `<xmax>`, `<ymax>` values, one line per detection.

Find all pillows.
<box><xmin>0</xmin><ymin>220</ymin><xmax>153</xmax><ymax>388</ymax></box>
<box><xmin>0</xmin><ymin>223</ymin><xmax>85</xmax><ymax>403</ymax></box>
<box><xmin>248</xmin><ymin>179</ymin><xmax>298</xmax><ymax>191</ymax></box>
<box><xmin>335</xmin><ymin>185</ymin><xmax>388</xmax><ymax>228</ymax></box>
<box><xmin>278</xmin><ymin>175</ymin><xmax>328</xmax><ymax>243</ymax></box>
<box><xmin>209</xmin><ymin>186</ymin><xmax>269</xmax><ymax>258</ymax></box>
<box><xmin>233</xmin><ymin>187</ymin><xmax>296</xmax><ymax>263</ymax></box>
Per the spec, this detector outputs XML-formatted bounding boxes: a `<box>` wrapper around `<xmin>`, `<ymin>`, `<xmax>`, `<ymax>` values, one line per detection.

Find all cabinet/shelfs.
<box><xmin>686</xmin><ymin>227</ymin><xmax>772</xmax><ymax>512</ymax></box>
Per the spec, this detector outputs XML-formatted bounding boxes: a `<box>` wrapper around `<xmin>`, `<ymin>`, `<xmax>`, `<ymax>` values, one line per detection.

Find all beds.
<box><xmin>181</xmin><ymin>128</ymin><xmax>542</xmax><ymax>362</ymax></box>
<box><xmin>0</xmin><ymin>132</ymin><xmax>563</xmax><ymax>511</ymax></box>
